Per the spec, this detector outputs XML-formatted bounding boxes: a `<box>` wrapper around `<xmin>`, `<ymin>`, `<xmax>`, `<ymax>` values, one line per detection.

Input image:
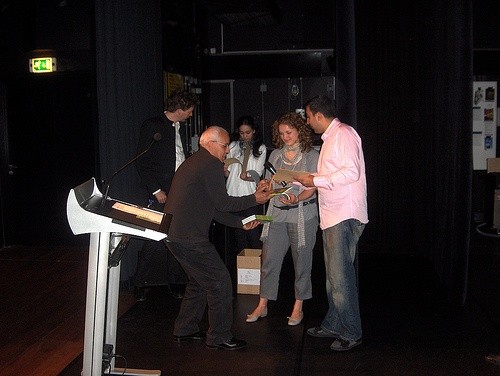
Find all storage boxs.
<box><xmin>237</xmin><ymin>248</ymin><xmax>263</xmax><ymax>295</ymax></box>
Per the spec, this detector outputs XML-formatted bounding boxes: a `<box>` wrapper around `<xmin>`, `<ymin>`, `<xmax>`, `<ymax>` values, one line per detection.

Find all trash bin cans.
<box><xmin>480</xmin><ymin>172</ymin><xmax>500</xmax><ymax>234</ymax></box>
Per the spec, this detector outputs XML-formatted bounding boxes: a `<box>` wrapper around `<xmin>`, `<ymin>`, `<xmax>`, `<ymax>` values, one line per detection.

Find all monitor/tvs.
<box><xmin>105</xmin><ymin>199</ymin><xmax>166</xmax><ymax>232</ymax></box>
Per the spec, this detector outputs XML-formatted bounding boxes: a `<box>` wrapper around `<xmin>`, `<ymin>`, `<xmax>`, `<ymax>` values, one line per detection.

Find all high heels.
<box><xmin>246</xmin><ymin>308</ymin><xmax>268</xmax><ymax>322</ymax></box>
<box><xmin>288</xmin><ymin>311</ymin><xmax>304</xmax><ymax>326</ymax></box>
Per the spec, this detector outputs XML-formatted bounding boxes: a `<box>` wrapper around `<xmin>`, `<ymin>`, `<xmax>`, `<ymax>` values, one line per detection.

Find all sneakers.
<box><xmin>330</xmin><ymin>337</ymin><xmax>363</xmax><ymax>351</ymax></box>
<box><xmin>307</xmin><ymin>326</ymin><xmax>339</xmax><ymax>337</ymax></box>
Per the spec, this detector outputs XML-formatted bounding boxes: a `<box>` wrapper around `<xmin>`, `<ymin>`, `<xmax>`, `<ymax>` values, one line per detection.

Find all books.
<box><xmin>267</xmin><ymin>184</ymin><xmax>293</xmax><ymax>202</ymax></box>
<box><xmin>241</xmin><ymin>212</ymin><xmax>273</xmax><ymax>226</ymax></box>
<box><xmin>271</xmin><ymin>167</ymin><xmax>311</xmax><ymax>185</ymax></box>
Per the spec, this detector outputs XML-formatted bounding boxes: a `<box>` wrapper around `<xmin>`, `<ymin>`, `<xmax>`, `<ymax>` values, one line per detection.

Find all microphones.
<box><xmin>103</xmin><ymin>132</ymin><xmax>163</xmax><ymax>199</ymax></box>
<box><xmin>264</xmin><ymin>161</ymin><xmax>287</xmax><ymax>187</ymax></box>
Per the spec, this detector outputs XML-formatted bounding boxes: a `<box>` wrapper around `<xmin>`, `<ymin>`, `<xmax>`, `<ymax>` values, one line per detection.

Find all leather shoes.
<box><xmin>173</xmin><ymin>331</ymin><xmax>209</xmax><ymax>344</ymax></box>
<box><xmin>133</xmin><ymin>286</ymin><xmax>149</xmax><ymax>301</ymax></box>
<box><xmin>173</xmin><ymin>283</ymin><xmax>188</xmax><ymax>299</ymax></box>
<box><xmin>207</xmin><ymin>337</ymin><xmax>247</xmax><ymax>351</ymax></box>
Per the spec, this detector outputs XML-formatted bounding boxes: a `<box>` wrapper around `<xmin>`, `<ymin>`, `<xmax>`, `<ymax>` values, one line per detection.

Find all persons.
<box><xmin>274</xmin><ymin>94</ymin><xmax>370</xmax><ymax>354</ymax></box>
<box><xmin>128</xmin><ymin>84</ymin><xmax>199</xmax><ymax>300</ymax></box>
<box><xmin>243</xmin><ymin>112</ymin><xmax>324</xmax><ymax>325</ymax></box>
<box><xmin>220</xmin><ymin>115</ymin><xmax>270</xmax><ymax>249</ymax></box>
<box><xmin>156</xmin><ymin>122</ymin><xmax>279</xmax><ymax>352</ymax></box>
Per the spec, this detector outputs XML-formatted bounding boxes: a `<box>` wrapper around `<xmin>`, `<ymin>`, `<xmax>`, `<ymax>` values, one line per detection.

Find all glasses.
<box><xmin>207</xmin><ymin>141</ymin><xmax>233</xmax><ymax>149</ymax></box>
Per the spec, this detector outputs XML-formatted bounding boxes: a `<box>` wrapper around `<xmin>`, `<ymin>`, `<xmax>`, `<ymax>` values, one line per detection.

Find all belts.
<box><xmin>272</xmin><ymin>198</ymin><xmax>316</xmax><ymax>210</ymax></box>
